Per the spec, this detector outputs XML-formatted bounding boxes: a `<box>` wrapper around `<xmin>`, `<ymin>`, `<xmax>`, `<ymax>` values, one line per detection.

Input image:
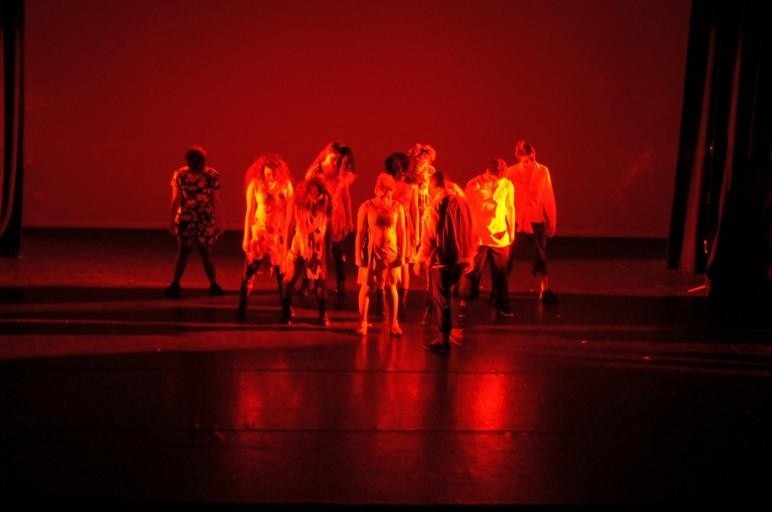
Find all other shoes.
<box><xmin>166</xmin><ymin>287</ymin><xmax>181</xmax><ymax>297</ymax></box>
<box><xmin>319</xmin><ymin>315</ymin><xmax>333</xmax><ymax>329</ymax></box>
<box><xmin>335</xmin><ymin>284</ymin><xmax>346</xmax><ymax>296</ymax></box>
<box><xmin>237</xmin><ymin>304</ymin><xmax>249</xmax><ymax>322</ymax></box>
<box><xmin>422</xmin><ymin>309</ymin><xmax>432</xmax><ymax>326</ymax></box>
<box><xmin>282</xmin><ymin>306</ymin><xmax>294</xmax><ymax>325</ymax></box>
<box><xmin>457</xmin><ymin>301</ymin><xmax>470</xmax><ymax>319</ymax></box>
<box><xmin>531</xmin><ymin>285</ymin><xmax>545</xmax><ymax>296</ymax></box>
<box><xmin>210</xmin><ymin>285</ymin><xmax>226</xmax><ymax>296</ymax></box>
<box><xmin>386</xmin><ymin>323</ymin><xmax>403</xmax><ymax>338</ymax></box>
<box><xmin>494</xmin><ymin>299</ymin><xmax>513</xmax><ymax>315</ymax></box>
<box><xmin>356</xmin><ymin>321</ymin><xmax>368</xmax><ymax>334</ymax></box>
<box><xmin>424</xmin><ymin>337</ymin><xmax>452</xmax><ymax>351</ymax></box>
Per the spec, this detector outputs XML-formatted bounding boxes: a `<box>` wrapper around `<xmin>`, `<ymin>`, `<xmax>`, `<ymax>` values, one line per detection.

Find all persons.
<box><xmin>164</xmin><ymin>146</ymin><xmax>225</xmax><ymax>296</ymax></box>
<box><xmin>413</xmin><ymin>171</ymin><xmax>472</xmax><ymax>349</ymax></box>
<box><xmin>235</xmin><ymin>142</ymin><xmax>358</xmax><ymax>329</ymax></box>
<box><xmin>457</xmin><ymin>159</ymin><xmax>516</xmax><ymax>326</ymax></box>
<box><xmin>504</xmin><ymin>140</ymin><xmax>557</xmax><ymax>313</ymax></box>
<box><xmin>358</xmin><ymin>143</ymin><xmax>436</xmax><ymax>338</ymax></box>
